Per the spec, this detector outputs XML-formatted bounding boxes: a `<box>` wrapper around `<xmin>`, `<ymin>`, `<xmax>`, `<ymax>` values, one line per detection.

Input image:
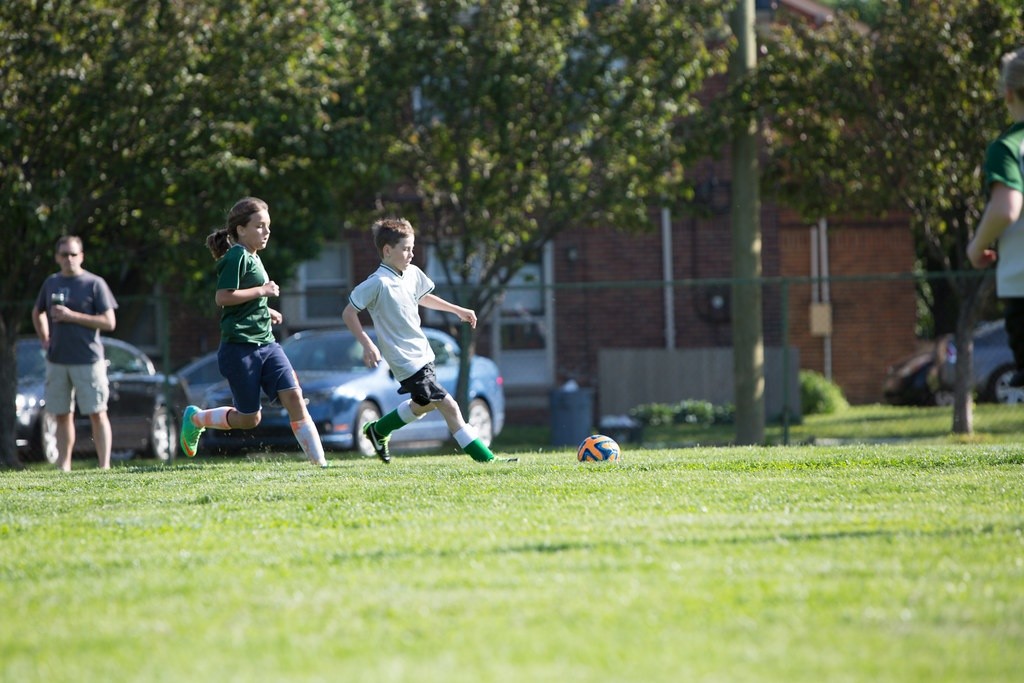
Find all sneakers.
<box><xmin>489</xmin><ymin>456</ymin><xmax>520</xmax><ymax>462</ymax></box>
<box><xmin>362</xmin><ymin>420</ymin><xmax>391</xmax><ymax>464</ymax></box>
<box><xmin>180</xmin><ymin>405</ymin><xmax>206</xmax><ymax>458</ymax></box>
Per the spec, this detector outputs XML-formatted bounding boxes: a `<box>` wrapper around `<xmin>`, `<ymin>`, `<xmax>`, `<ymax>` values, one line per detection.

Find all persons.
<box><xmin>966</xmin><ymin>48</ymin><xmax>1024</xmax><ymax>387</ymax></box>
<box><xmin>32</xmin><ymin>236</ymin><xmax>118</xmax><ymax>470</ymax></box>
<box><xmin>180</xmin><ymin>197</ymin><xmax>327</xmax><ymax>466</ymax></box>
<box><xmin>342</xmin><ymin>218</ymin><xmax>519</xmax><ymax>465</ymax></box>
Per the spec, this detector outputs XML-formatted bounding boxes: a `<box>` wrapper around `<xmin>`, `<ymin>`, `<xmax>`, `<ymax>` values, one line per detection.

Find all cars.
<box><xmin>881</xmin><ymin>318</ymin><xmax>1024</xmax><ymax>404</ymax></box>
<box><xmin>13</xmin><ymin>336</ymin><xmax>227</xmax><ymax>460</ymax></box>
<box><xmin>202</xmin><ymin>327</ymin><xmax>506</xmax><ymax>457</ymax></box>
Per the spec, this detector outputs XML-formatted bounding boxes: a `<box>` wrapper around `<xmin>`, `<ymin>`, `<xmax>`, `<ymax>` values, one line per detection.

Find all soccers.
<box><xmin>577</xmin><ymin>434</ymin><xmax>621</xmax><ymax>464</ymax></box>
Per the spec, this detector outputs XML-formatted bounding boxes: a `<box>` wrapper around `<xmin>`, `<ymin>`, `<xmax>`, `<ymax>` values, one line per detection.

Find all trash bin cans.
<box><xmin>599</xmin><ymin>425</ymin><xmax>643</xmax><ymax>445</ymax></box>
<box><xmin>549</xmin><ymin>386</ymin><xmax>597</xmax><ymax>448</ymax></box>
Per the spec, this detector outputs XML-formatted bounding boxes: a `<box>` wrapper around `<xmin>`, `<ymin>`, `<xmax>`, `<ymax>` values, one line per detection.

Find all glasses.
<box><xmin>56</xmin><ymin>253</ymin><xmax>78</xmax><ymax>257</ymax></box>
